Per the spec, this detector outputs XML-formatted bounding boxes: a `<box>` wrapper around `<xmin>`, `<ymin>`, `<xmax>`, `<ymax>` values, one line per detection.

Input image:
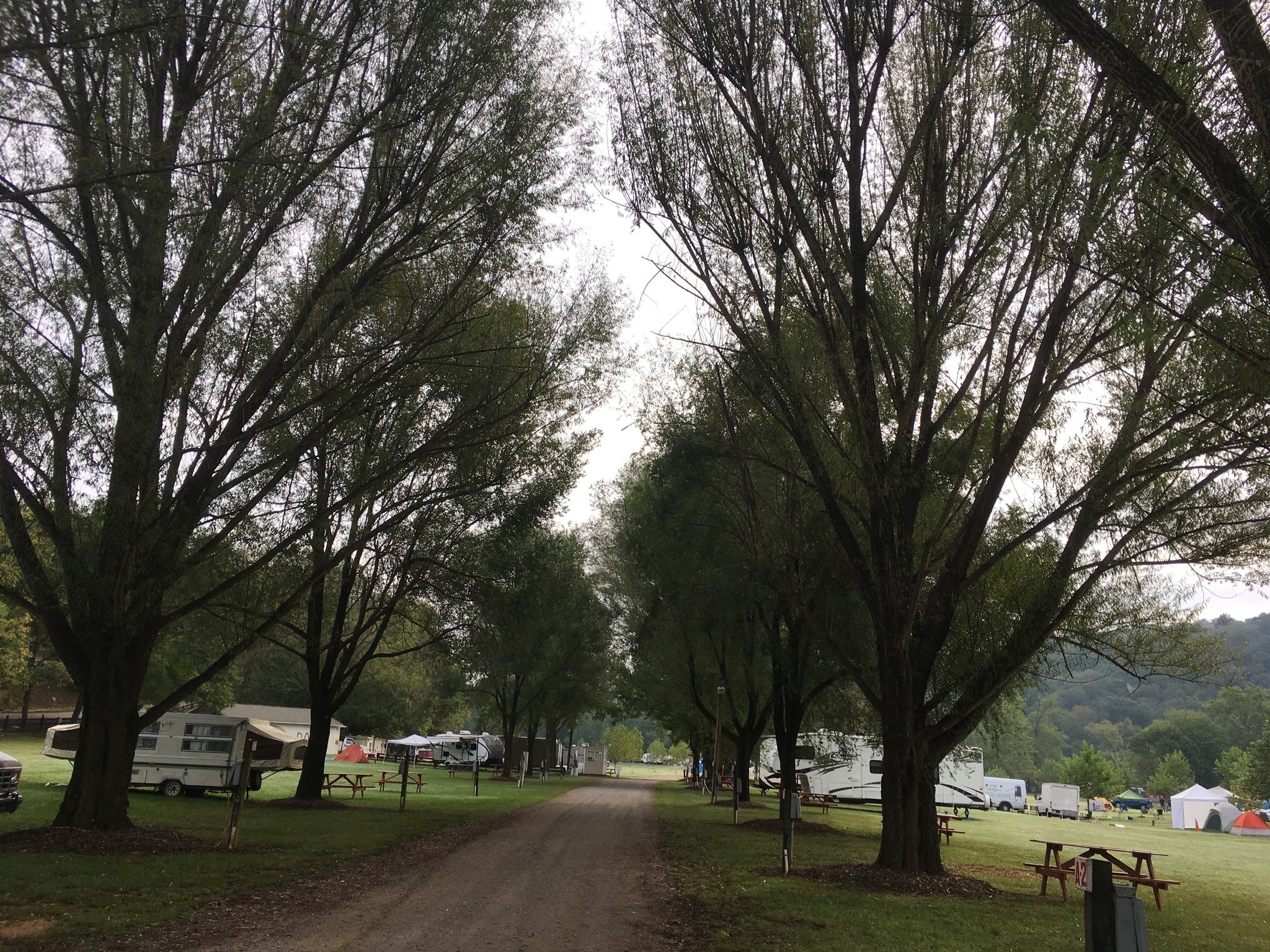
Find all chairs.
<box><xmin>1027</xmin><ymin>805</ymin><xmax>1039</xmax><ymax>816</ymax></box>
<box><xmin>1157</xmin><ymin>809</ymin><xmax>1163</xmax><ymax>820</ymax></box>
<box><xmin>1080</xmin><ymin>810</ymin><xmax>1100</xmax><ymax>824</ymax></box>
<box><xmin>1120</xmin><ymin>805</ymin><xmax>1128</xmax><ymax>813</ymax></box>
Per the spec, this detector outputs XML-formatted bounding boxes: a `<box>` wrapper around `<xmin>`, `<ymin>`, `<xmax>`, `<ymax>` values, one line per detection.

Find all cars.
<box><xmin>0</xmin><ymin>752</ymin><xmax>24</xmax><ymax>814</ymax></box>
<box><xmin>1110</xmin><ymin>799</ymin><xmax>1152</xmax><ymax>810</ymax></box>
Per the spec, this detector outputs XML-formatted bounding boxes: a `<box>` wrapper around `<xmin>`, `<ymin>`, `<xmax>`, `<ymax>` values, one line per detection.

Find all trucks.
<box><xmin>758</xmin><ymin>726</ymin><xmax>991</xmax><ymax>820</ymax></box>
<box><xmin>1036</xmin><ymin>782</ymin><xmax>1080</xmax><ymax>820</ymax></box>
<box><xmin>41</xmin><ymin>711</ymin><xmax>308</xmax><ymax>798</ymax></box>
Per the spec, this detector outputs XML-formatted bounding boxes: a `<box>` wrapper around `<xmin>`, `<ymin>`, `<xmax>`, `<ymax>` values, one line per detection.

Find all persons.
<box><xmin>1159</xmin><ymin>796</ymin><xmax>1166</xmax><ymax>813</ymax></box>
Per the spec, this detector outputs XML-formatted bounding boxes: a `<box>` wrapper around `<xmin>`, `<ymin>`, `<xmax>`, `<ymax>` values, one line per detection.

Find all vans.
<box><xmin>984</xmin><ymin>777</ymin><xmax>1029</xmax><ymax>813</ymax></box>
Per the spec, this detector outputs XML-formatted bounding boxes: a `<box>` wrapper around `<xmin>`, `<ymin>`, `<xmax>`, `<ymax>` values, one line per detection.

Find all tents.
<box><xmin>383</xmin><ymin>734</ymin><xmax>432</xmax><ymax>768</ymax></box>
<box><xmin>1085</xmin><ymin>783</ymin><xmax>1270</xmax><ymax>836</ymax></box>
<box><xmin>334</xmin><ymin>745</ymin><xmax>369</xmax><ymax>764</ymax></box>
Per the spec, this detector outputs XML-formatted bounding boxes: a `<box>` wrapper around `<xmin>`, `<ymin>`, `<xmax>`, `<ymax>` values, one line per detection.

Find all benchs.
<box><xmin>446</xmin><ymin>770</ymin><xmax>474</xmax><ymax>778</ymax></box>
<box><xmin>937</xmin><ymin>828</ymin><xmax>965</xmax><ymax>846</ymax></box>
<box><xmin>1059</xmin><ymin>809</ymin><xmax>1080</xmax><ymax>822</ymax></box>
<box><xmin>801</xmin><ymin>801</ymin><xmax>838</xmax><ymax>815</ymax></box>
<box><xmin>1023</xmin><ymin>863</ymin><xmax>1180</xmax><ymax>911</ymax></box>
<box><xmin>368</xmin><ymin>758</ymin><xmax>377</xmax><ymax>764</ymax></box>
<box><xmin>322</xmin><ymin>780</ymin><xmax>427</xmax><ymax>800</ymax></box>
<box><xmin>491</xmin><ymin>770</ymin><xmax>514</xmax><ymax>777</ymax></box>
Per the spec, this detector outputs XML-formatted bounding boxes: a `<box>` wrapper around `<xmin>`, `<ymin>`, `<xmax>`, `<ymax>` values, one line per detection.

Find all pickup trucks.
<box><xmin>338</xmin><ymin>738</ymin><xmax>372</xmax><ymax>757</ymax></box>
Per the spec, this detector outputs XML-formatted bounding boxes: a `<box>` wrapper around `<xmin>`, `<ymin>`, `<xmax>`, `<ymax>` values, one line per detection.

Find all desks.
<box><xmin>798</xmin><ymin>792</ymin><xmax>837</xmax><ymax>815</ymax></box>
<box><xmin>365</xmin><ymin>753</ymin><xmax>376</xmax><ymax>764</ymax></box>
<box><xmin>490</xmin><ymin>764</ymin><xmax>515</xmax><ymax>777</ymax></box>
<box><xmin>448</xmin><ymin>764</ymin><xmax>474</xmax><ymax>778</ymax></box>
<box><xmin>322</xmin><ymin>773</ymin><xmax>376</xmax><ymax>800</ymax></box>
<box><xmin>937</xmin><ymin>813</ymin><xmax>962</xmax><ymax>845</ymax></box>
<box><xmin>376</xmin><ymin>770</ymin><xmax>426</xmax><ymax>793</ymax></box>
<box><xmin>1029</xmin><ymin>839</ymin><xmax>1169</xmax><ymax>911</ymax></box>
<box><xmin>1103</xmin><ymin>808</ymin><xmax>1122</xmax><ymax>820</ymax></box>
